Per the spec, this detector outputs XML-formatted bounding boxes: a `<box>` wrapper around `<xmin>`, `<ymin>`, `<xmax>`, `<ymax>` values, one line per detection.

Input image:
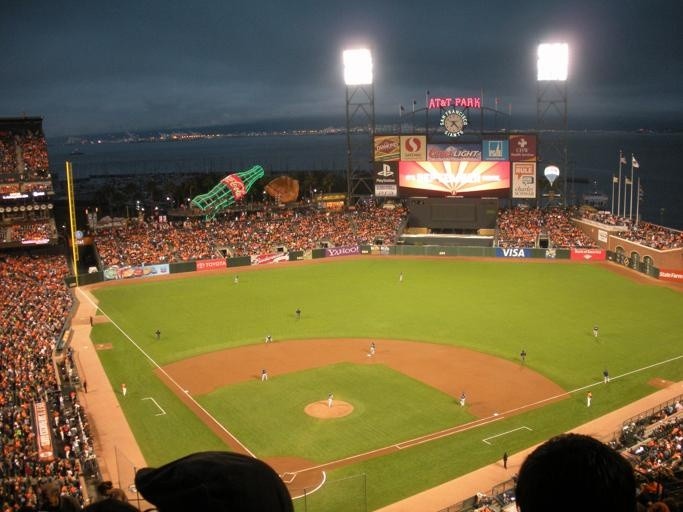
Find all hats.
<box><xmin>135</xmin><ymin>451</ymin><xmax>295</xmax><ymax>512</ymax></box>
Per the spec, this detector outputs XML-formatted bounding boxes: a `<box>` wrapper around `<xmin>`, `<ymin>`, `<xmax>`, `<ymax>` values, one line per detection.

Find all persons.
<box><xmin>369</xmin><ymin>341</ymin><xmax>375</xmax><ymax>354</ymax></box>
<box><xmin>459</xmin><ymin>393</ymin><xmax>466</xmax><ymax>406</ymax></box>
<box><xmin>143</xmin><ymin>201</ymin><xmax>410</xmax><ymax>263</ymax></box>
<box><xmin>264</xmin><ymin>335</ymin><xmax>273</xmax><ymax>343</ymax></box>
<box><xmin>0</xmin><ymin>125</ymin><xmax>142</xmax><ymax>511</ymax></box>
<box><xmin>496</xmin><ymin>203</ymin><xmax>681</xmax><ymax>251</ymax></box>
<box><xmin>593</xmin><ymin>324</ymin><xmax>599</xmax><ymax>340</ymax></box>
<box><xmin>154</xmin><ymin>329</ymin><xmax>162</xmax><ymax>338</ymax></box>
<box><xmin>327</xmin><ymin>391</ymin><xmax>334</xmax><ymax>408</ymax></box>
<box><xmin>398</xmin><ymin>271</ymin><xmax>403</xmax><ymax>282</ymax></box>
<box><xmin>260</xmin><ymin>367</ymin><xmax>267</xmax><ymax>381</ymax></box>
<box><xmin>519</xmin><ymin>349</ymin><xmax>528</xmax><ymax>362</ymax></box>
<box><xmin>473</xmin><ymin>401</ymin><xmax>683</xmax><ymax>512</ymax></box>
<box><xmin>587</xmin><ymin>390</ymin><xmax>592</xmax><ymax>407</ymax></box>
<box><xmin>234</xmin><ymin>275</ymin><xmax>239</xmax><ymax>282</ymax></box>
<box><xmin>133</xmin><ymin>451</ymin><xmax>292</xmax><ymax>511</ymax></box>
<box><xmin>295</xmin><ymin>307</ymin><xmax>301</xmax><ymax>319</ymax></box>
<box><xmin>603</xmin><ymin>368</ymin><xmax>609</xmax><ymax>385</ymax></box>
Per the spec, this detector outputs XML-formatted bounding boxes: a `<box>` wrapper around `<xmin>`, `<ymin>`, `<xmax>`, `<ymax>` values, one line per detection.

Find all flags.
<box><xmin>611</xmin><ymin>151</ymin><xmax>647</xmax><ymax>203</ymax></box>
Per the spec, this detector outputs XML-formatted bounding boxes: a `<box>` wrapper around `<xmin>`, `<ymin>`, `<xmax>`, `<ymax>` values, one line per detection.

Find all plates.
<box><xmin>103</xmin><ymin>266</ymin><xmax>152</xmax><ymax>280</ymax></box>
